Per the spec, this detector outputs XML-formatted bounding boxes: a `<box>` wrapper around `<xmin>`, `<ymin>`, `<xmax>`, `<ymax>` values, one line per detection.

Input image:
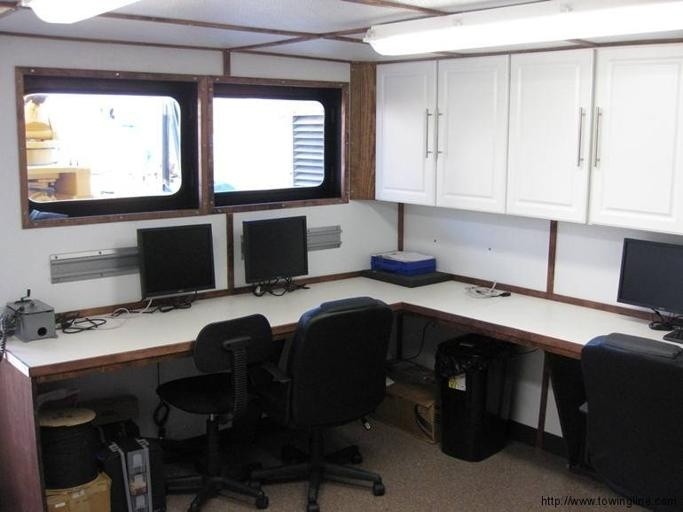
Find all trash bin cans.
<box><xmin>434</xmin><ymin>335</ymin><xmax>512</xmax><ymax>459</ymax></box>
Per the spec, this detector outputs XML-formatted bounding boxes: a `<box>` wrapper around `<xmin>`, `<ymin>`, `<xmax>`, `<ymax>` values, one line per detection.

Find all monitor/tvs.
<box><xmin>615</xmin><ymin>239</ymin><xmax>680</xmax><ymax>316</ymax></box>
<box><xmin>242</xmin><ymin>216</ymin><xmax>308</xmax><ymax>284</ymax></box>
<box><xmin>136</xmin><ymin>223</ymin><xmax>215</xmax><ymax>301</ymax></box>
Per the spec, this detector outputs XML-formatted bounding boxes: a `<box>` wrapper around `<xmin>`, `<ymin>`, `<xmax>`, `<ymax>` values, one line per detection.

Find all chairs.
<box><xmin>576</xmin><ymin>331</ymin><xmax>683</xmax><ymax>508</ymax></box>
<box><xmin>149</xmin><ymin>310</ymin><xmax>286</xmax><ymax>508</ymax></box>
<box><xmin>254</xmin><ymin>294</ymin><xmax>396</xmax><ymax>507</ymax></box>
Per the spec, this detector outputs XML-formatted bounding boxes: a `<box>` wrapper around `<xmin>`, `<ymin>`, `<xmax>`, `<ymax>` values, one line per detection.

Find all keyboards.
<box><xmin>661</xmin><ymin>327</ymin><xmax>681</xmax><ymax>346</ymax></box>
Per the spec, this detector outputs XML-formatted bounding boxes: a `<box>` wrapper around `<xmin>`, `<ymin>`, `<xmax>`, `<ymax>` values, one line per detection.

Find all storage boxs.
<box><xmin>43</xmin><ymin>474</ymin><xmax>113</xmax><ymax>511</ymax></box>
<box><xmin>380</xmin><ymin>358</ymin><xmax>441</xmax><ymax>444</ymax></box>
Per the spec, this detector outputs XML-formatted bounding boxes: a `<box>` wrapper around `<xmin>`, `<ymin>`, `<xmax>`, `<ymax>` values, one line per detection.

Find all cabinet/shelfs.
<box><xmin>373</xmin><ymin>52</ymin><xmax>510</xmax><ymax>213</ymax></box>
<box><xmin>505</xmin><ymin>40</ymin><xmax>683</xmax><ymax>243</ymax></box>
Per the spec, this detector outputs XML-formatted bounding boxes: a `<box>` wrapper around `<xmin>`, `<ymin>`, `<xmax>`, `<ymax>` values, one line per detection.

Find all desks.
<box><xmin>1</xmin><ymin>270</ymin><xmax>683</xmax><ymax>507</ymax></box>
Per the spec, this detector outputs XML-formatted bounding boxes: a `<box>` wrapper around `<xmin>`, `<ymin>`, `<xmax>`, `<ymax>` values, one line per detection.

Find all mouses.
<box><xmin>648</xmin><ymin>321</ymin><xmax>668</xmax><ymax>331</ymax></box>
<box><xmin>253</xmin><ymin>285</ymin><xmax>264</xmax><ymax>296</ymax></box>
